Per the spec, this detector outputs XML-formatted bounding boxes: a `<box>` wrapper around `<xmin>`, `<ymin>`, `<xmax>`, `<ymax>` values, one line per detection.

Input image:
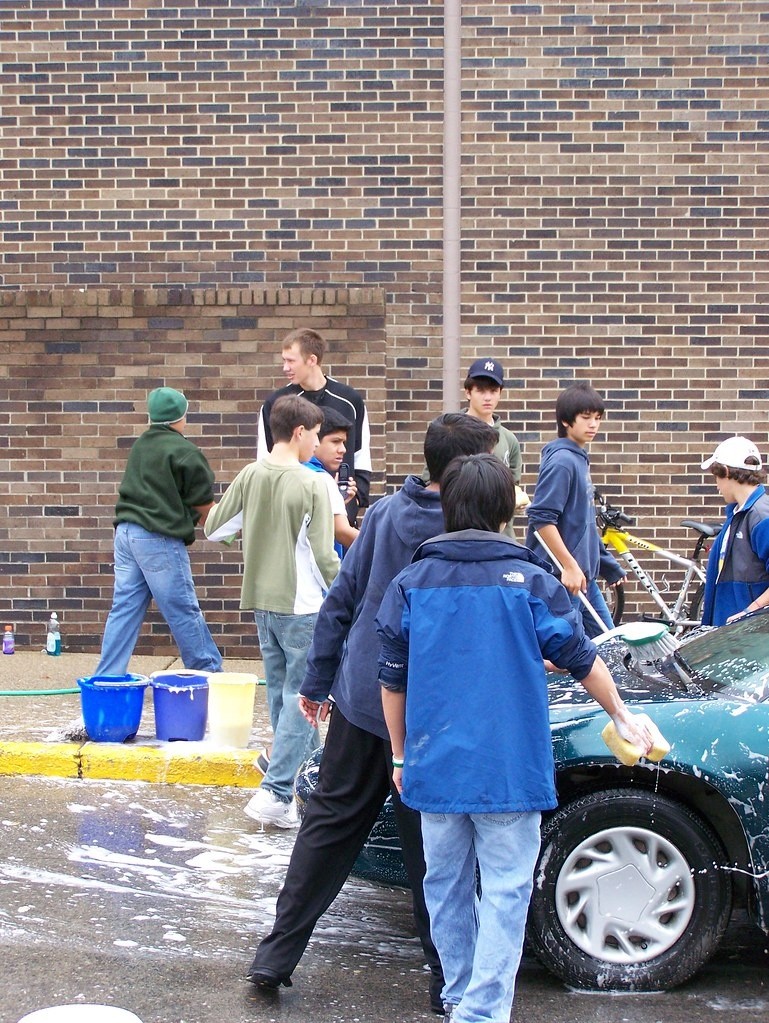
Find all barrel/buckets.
<box><xmin>149</xmin><ymin>669</ymin><xmax>212</xmax><ymax>741</ymax></box>
<box><xmin>206</xmin><ymin>672</ymin><xmax>258</xmax><ymax>748</ymax></box>
<box><xmin>75</xmin><ymin>673</ymin><xmax>151</xmax><ymax>743</ymax></box>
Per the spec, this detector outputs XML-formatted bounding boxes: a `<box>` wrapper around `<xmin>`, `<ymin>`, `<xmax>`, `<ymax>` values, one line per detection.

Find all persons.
<box><xmin>422</xmin><ymin>359</ymin><xmax>531</xmax><ymax>543</ymax></box>
<box><xmin>701</xmin><ymin>437</ymin><xmax>769</xmax><ymax>627</ymax></box>
<box><xmin>374</xmin><ymin>453</ymin><xmax>654</xmax><ymax>1023</ymax></box>
<box><xmin>204</xmin><ymin>328</ymin><xmax>373</xmax><ymax>829</ymax></box>
<box><xmin>526</xmin><ymin>382</ymin><xmax>628</xmax><ymax>641</ymax></box>
<box><xmin>94</xmin><ymin>387</ymin><xmax>224</xmax><ymax>674</ymax></box>
<box><xmin>246</xmin><ymin>413</ymin><xmax>568</xmax><ymax>987</ymax></box>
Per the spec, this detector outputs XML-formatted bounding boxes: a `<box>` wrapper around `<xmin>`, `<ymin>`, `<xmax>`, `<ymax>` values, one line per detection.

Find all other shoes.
<box><xmin>430</xmin><ymin>995</ymin><xmax>445</xmax><ymax>1014</ymax></box>
<box><xmin>442</xmin><ymin>1004</ymin><xmax>458</xmax><ymax>1023</ymax></box>
<box><xmin>245</xmin><ymin>967</ymin><xmax>277</xmax><ymax>985</ymax></box>
<box><xmin>244</xmin><ymin>788</ymin><xmax>302</xmax><ymax>829</ymax></box>
<box><xmin>254</xmin><ymin>747</ymin><xmax>307</xmax><ymax>806</ymax></box>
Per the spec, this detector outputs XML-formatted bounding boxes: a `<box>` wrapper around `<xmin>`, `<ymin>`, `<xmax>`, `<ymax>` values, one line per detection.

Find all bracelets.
<box><xmin>392</xmin><ymin>762</ymin><xmax>403</xmax><ymax>768</ymax></box>
<box><xmin>392</xmin><ymin>755</ymin><xmax>404</xmax><ymax>764</ymax></box>
<box><xmin>744</xmin><ymin>608</ymin><xmax>750</xmax><ymax>614</ymax></box>
<box><xmin>755</xmin><ymin>600</ymin><xmax>762</xmax><ymax>608</ymax></box>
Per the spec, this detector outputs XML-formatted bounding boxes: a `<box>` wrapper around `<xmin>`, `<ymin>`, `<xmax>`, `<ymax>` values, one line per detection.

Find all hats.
<box><xmin>701</xmin><ymin>436</ymin><xmax>762</xmax><ymax>471</ymax></box>
<box><xmin>147</xmin><ymin>387</ymin><xmax>189</xmax><ymax>424</ymax></box>
<box><xmin>467</xmin><ymin>358</ymin><xmax>504</xmax><ymax>386</ymax></box>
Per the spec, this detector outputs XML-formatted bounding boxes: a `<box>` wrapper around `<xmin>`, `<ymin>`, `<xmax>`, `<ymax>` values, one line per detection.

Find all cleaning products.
<box><xmin>2</xmin><ymin>625</ymin><xmax>15</xmax><ymax>655</ymax></box>
<box><xmin>46</xmin><ymin>612</ymin><xmax>61</xmax><ymax>657</ymax></box>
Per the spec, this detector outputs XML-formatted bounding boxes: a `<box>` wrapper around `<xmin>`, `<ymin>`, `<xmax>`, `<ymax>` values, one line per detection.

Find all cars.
<box><xmin>291</xmin><ymin>602</ymin><xmax>769</xmax><ymax>999</ymax></box>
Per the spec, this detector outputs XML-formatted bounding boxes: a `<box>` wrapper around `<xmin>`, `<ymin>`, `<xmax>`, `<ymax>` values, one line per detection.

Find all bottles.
<box><xmin>2</xmin><ymin>626</ymin><xmax>15</xmax><ymax>654</ymax></box>
<box><xmin>46</xmin><ymin>612</ymin><xmax>62</xmax><ymax>656</ymax></box>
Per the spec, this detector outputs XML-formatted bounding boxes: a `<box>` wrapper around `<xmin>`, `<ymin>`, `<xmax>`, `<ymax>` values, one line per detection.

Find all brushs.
<box><xmin>589</xmin><ymin>621</ymin><xmax>682</xmax><ymax>662</ymax></box>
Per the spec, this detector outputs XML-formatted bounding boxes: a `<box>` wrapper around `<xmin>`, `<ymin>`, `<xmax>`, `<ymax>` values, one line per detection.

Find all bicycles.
<box><xmin>591</xmin><ymin>492</ymin><xmax>725</xmax><ymax>638</ymax></box>
